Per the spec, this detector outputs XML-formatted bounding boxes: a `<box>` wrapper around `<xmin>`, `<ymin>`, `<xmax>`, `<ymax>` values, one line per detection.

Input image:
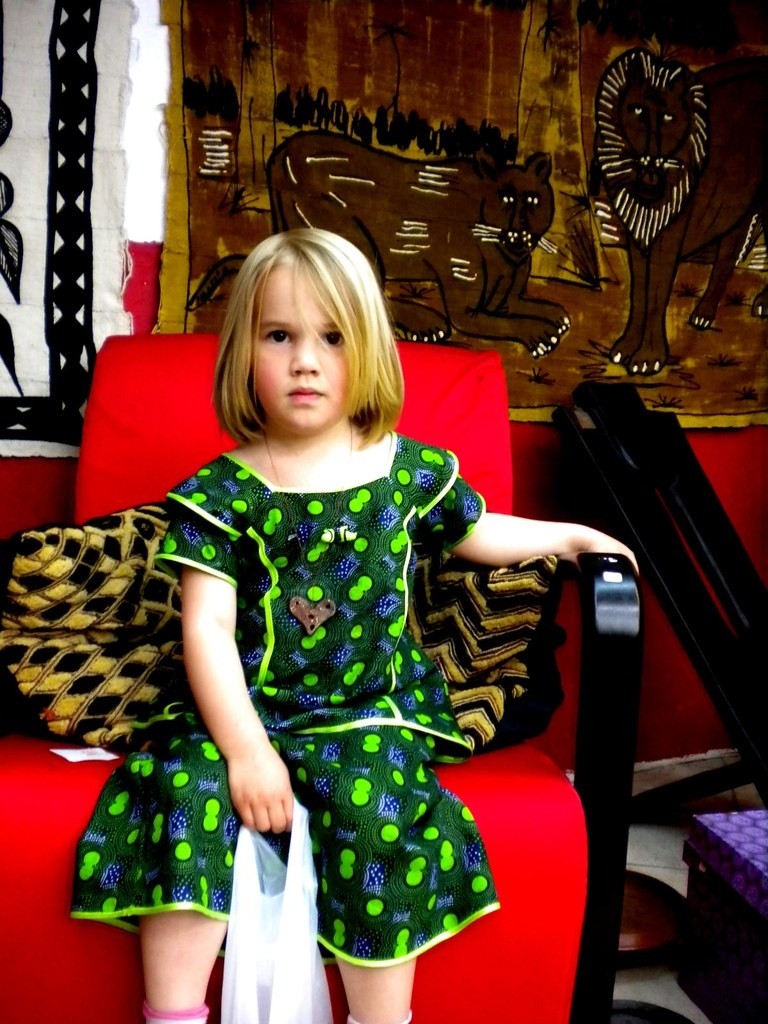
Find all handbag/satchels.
<box><xmin>220</xmin><ymin>797</ymin><xmax>333</xmax><ymax>1024</ymax></box>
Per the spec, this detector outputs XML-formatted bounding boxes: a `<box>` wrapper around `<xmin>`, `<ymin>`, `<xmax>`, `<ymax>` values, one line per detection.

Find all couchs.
<box><xmin>0</xmin><ymin>333</ymin><xmax>643</xmax><ymax>1024</ymax></box>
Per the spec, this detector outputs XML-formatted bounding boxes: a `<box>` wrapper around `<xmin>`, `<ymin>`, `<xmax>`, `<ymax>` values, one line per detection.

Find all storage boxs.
<box><xmin>682</xmin><ymin>810</ymin><xmax>768</xmax><ymax>1024</ymax></box>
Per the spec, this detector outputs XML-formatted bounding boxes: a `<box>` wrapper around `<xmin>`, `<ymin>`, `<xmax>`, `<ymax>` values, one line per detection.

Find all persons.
<box><xmin>71</xmin><ymin>228</ymin><xmax>640</xmax><ymax>1024</ymax></box>
